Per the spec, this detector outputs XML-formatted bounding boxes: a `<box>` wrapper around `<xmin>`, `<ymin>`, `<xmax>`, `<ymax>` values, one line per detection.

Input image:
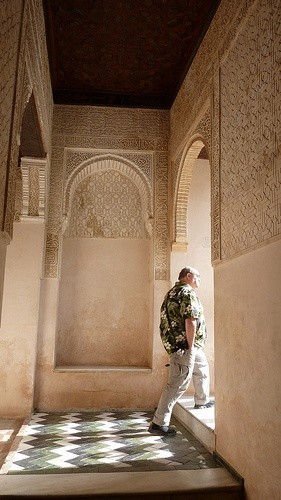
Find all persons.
<box><xmin>148</xmin><ymin>266</ymin><xmax>215</xmax><ymax>437</ymax></box>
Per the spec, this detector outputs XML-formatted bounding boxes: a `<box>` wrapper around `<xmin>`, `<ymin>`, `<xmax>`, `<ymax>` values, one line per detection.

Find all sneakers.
<box><xmin>148</xmin><ymin>422</ymin><xmax>176</xmax><ymax>438</ymax></box>
<box><xmin>195</xmin><ymin>400</ymin><xmax>214</xmax><ymax>408</ymax></box>
